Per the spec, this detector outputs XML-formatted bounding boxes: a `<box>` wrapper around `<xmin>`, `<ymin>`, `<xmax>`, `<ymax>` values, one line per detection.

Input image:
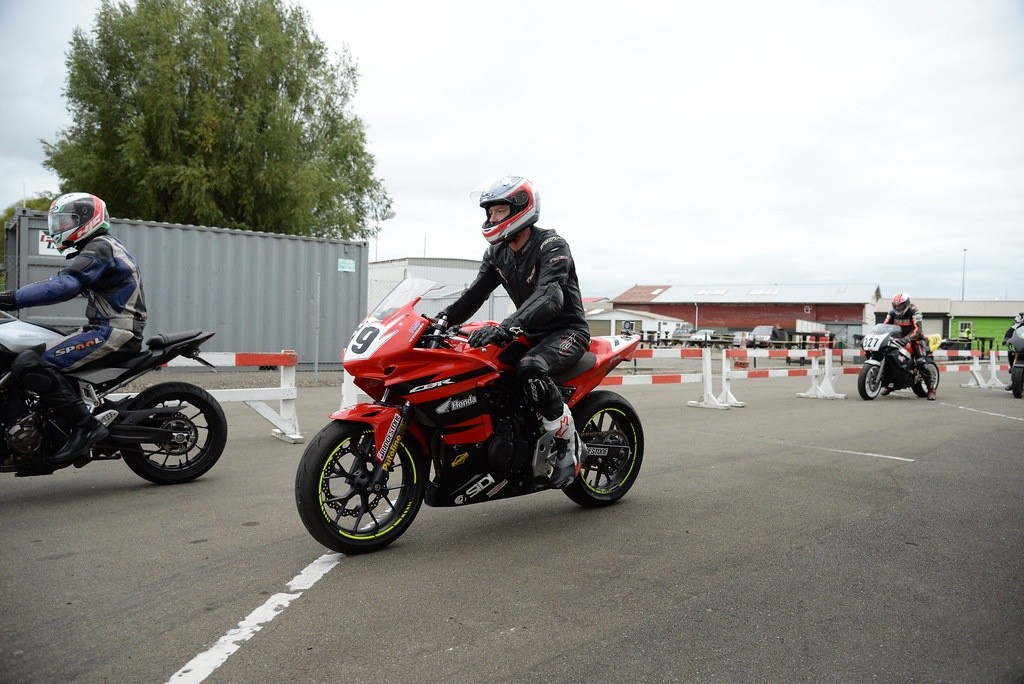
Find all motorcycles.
<box><xmin>1006</xmin><ymin>321</ymin><xmax>1024</xmax><ymax>397</ymax></box>
<box><xmin>293</xmin><ymin>277</ymin><xmax>644</xmax><ymax>556</ymax></box>
<box><xmin>855</xmin><ymin>320</ymin><xmax>940</xmax><ymax>400</ymax></box>
<box><xmin>1</xmin><ymin>306</ymin><xmax>227</xmax><ymax>484</ymax></box>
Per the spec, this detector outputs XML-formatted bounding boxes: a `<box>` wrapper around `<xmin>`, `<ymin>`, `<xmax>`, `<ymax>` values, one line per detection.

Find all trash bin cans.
<box><xmin>946</xmin><ymin>338</ymin><xmax>967</xmax><ymax>361</ymax></box>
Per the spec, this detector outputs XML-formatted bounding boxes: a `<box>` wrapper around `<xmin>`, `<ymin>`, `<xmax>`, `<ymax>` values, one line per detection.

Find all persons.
<box><xmin>422</xmin><ymin>174</ymin><xmax>592</xmax><ymax>489</ymax></box>
<box><xmin>1002</xmin><ymin>310</ymin><xmax>1023</xmax><ymax>392</ymax></box>
<box><xmin>880</xmin><ymin>293</ymin><xmax>937</xmax><ymax>400</ymax></box>
<box><xmin>1</xmin><ymin>193</ymin><xmax>147</xmax><ymax>461</ymax></box>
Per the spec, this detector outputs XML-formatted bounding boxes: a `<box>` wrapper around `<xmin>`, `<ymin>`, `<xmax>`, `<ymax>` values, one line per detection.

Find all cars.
<box><xmin>745</xmin><ymin>325</ymin><xmax>781</xmax><ymax>350</ymax></box>
<box><xmin>668</xmin><ymin>325</ymin><xmax>724</xmax><ymax>346</ymax></box>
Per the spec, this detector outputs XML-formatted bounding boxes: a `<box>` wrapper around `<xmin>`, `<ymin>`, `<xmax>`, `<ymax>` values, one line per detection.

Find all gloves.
<box><xmin>1002</xmin><ymin>339</ymin><xmax>1010</xmax><ymax>346</ymax></box>
<box><xmin>467</xmin><ymin>318</ymin><xmax>525</xmax><ymax>348</ymax></box>
<box><xmin>896</xmin><ymin>338</ymin><xmax>907</xmax><ymax>346</ymax></box>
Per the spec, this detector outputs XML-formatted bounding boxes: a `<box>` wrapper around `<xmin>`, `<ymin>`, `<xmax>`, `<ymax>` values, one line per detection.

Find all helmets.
<box><xmin>49</xmin><ymin>192</ymin><xmax>111</xmax><ymax>254</ymax></box>
<box><xmin>891</xmin><ymin>292</ymin><xmax>911</xmax><ymax>316</ymax></box>
<box><xmin>480</xmin><ymin>177</ymin><xmax>540</xmax><ymax>245</ymax></box>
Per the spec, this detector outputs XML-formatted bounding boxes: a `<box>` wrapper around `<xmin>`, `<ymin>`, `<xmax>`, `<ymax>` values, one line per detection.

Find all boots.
<box><xmin>880</xmin><ymin>389</ymin><xmax>891</xmax><ymax>396</ymax></box>
<box><xmin>925</xmin><ymin>380</ymin><xmax>937</xmax><ymax>400</ymax></box>
<box><xmin>1005</xmin><ymin>382</ymin><xmax>1013</xmax><ymax>391</ymax></box>
<box><xmin>53</xmin><ymin>396</ymin><xmax>110</xmax><ymax>461</ymax></box>
<box><xmin>543</xmin><ymin>402</ymin><xmax>589</xmax><ymax>489</ymax></box>
